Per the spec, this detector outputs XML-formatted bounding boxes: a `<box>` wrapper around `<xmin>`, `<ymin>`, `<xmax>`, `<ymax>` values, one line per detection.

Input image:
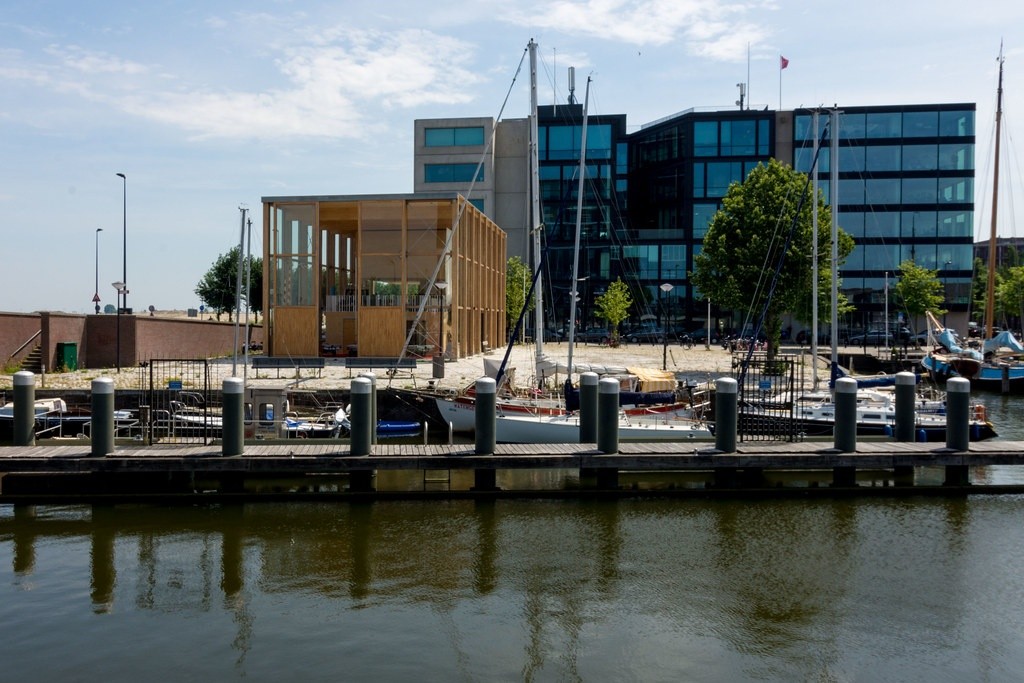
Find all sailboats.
<box><xmin>1</xmin><ymin>38</ymin><xmax>999</xmax><ymax>441</ymax></box>
<box><xmin>920</xmin><ymin>38</ymin><xmax>1024</xmax><ymax>392</ymax></box>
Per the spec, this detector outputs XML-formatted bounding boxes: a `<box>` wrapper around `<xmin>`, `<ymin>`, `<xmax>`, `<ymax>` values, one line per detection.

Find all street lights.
<box><xmin>95</xmin><ymin>228</ymin><xmax>104</xmax><ymax>314</ymax></box>
<box><xmin>116</xmin><ymin>172</ymin><xmax>127</xmax><ymax>313</ymax></box>
<box><xmin>111</xmin><ymin>281</ymin><xmax>126</xmax><ymax>373</ymax></box>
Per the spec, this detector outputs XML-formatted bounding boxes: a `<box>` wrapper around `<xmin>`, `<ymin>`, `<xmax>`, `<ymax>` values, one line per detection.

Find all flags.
<box><xmin>781</xmin><ymin>56</ymin><xmax>789</xmax><ymax>69</ymax></box>
